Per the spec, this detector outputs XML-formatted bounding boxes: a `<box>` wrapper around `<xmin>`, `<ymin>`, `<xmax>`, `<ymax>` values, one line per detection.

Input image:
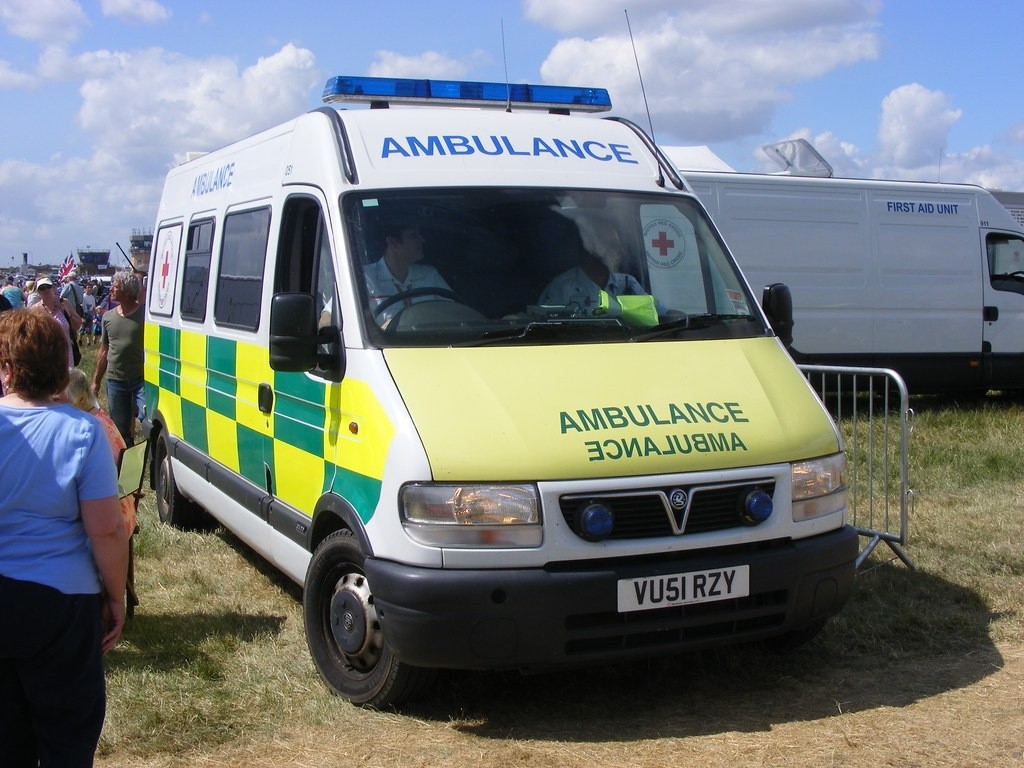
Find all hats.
<box><xmin>36</xmin><ymin>277</ymin><xmax>53</xmax><ymax>288</ymax></box>
<box><xmin>67</xmin><ymin>272</ymin><xmax>76</xmax><ymax>277</ymax></box>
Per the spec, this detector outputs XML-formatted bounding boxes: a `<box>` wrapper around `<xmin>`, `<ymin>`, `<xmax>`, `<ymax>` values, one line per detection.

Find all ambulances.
<box><xmin>140</xmin><ymin>74</ymin><xmax>858</xmax><ymax>707</ymax></box>
<box><xmin>638</xmin><ymin>137</ymin><xmax>1023</xmax><ymax>403</ymax></box>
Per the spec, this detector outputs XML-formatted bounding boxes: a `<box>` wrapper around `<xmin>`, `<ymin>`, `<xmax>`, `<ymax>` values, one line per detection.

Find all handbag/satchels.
<box><xmin>71</xmin><ymin>337</ymin><xmax>81</xmax><ymax>366</ymax></box>
<box><xmin>76</xmin><ymin>304</ymin><xmax>84</xmax><ymax>318</ymax></box>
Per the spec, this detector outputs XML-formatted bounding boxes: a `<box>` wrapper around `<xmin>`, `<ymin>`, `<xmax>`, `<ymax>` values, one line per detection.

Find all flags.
<box><xmin>57</xmin><ymin>250</ymin><xmax>75</xmax><ymax>284</ymax></box>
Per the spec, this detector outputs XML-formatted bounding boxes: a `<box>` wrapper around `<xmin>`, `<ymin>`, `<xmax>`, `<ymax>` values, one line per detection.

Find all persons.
<box><xmin>0</xmin><ymin>267</ymin><xmax>148</xmax><ymax>768</ymax></box>
<box><xmin>536</xmin><ymin>215</ymin><xmax>670</xmax><ymax>317</ymax></box>
<box><xmin>317</xmin><ymin>220</ymin><xmax>460</xmax><ymax>332</ymax></box>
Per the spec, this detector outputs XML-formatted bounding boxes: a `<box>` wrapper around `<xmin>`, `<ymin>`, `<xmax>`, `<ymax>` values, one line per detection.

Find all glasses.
<box><xmin>39</xmin><ymin>285</ymin><xmax>52</xmax><ymax>290</ymax></box>
<box><xmin>598</xmin><ymin>239</ymin><xmax>622</xmax><ymax>250</ymax></box>
